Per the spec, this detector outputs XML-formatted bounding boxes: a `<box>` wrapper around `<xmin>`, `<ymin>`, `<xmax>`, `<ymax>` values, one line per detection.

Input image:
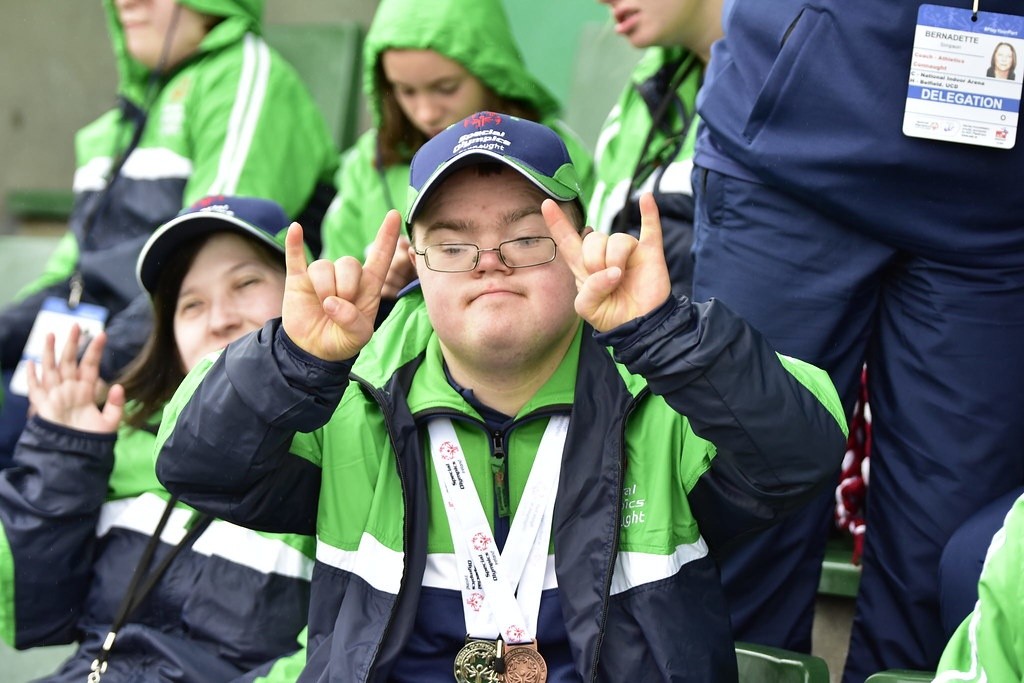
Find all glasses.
<box><xmin>414</xmin><ymin>236</ymin><xmax>557</xmax><ymax>273</ymax></box>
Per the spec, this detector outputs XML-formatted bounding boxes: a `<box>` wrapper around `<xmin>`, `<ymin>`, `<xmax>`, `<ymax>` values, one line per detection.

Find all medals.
<box><xmin>451</xmin><ymin>642</ymin><xmax>549</xmax><ymax>683</ymax></box>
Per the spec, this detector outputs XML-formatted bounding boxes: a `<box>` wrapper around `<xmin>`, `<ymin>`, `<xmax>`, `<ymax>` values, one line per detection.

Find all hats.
<box><xmin>136</xmin><ymin>195</ymin><xmax>290</xmax><ymax>299</ymax></box>
<box><xmin>404</xmin><ymin>110</ymin><xmax>587</xmax><ymax>226</ymax></box>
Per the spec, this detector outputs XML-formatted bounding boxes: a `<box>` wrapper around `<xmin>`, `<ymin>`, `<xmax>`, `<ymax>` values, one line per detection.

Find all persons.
<box><xmin>987</xmin><ymin>43</ymin><xmax>1017</xmax><ymax>80</ymax></box>
<box><xmin>153</xmin><ymin>111</ymin><xmax>851</xmax><ymax>683</ymax></box>
<box><xmin>0</xmin><ymin>0</ymin><xmax>1024</xmax><ymax>683</ymax></box>
